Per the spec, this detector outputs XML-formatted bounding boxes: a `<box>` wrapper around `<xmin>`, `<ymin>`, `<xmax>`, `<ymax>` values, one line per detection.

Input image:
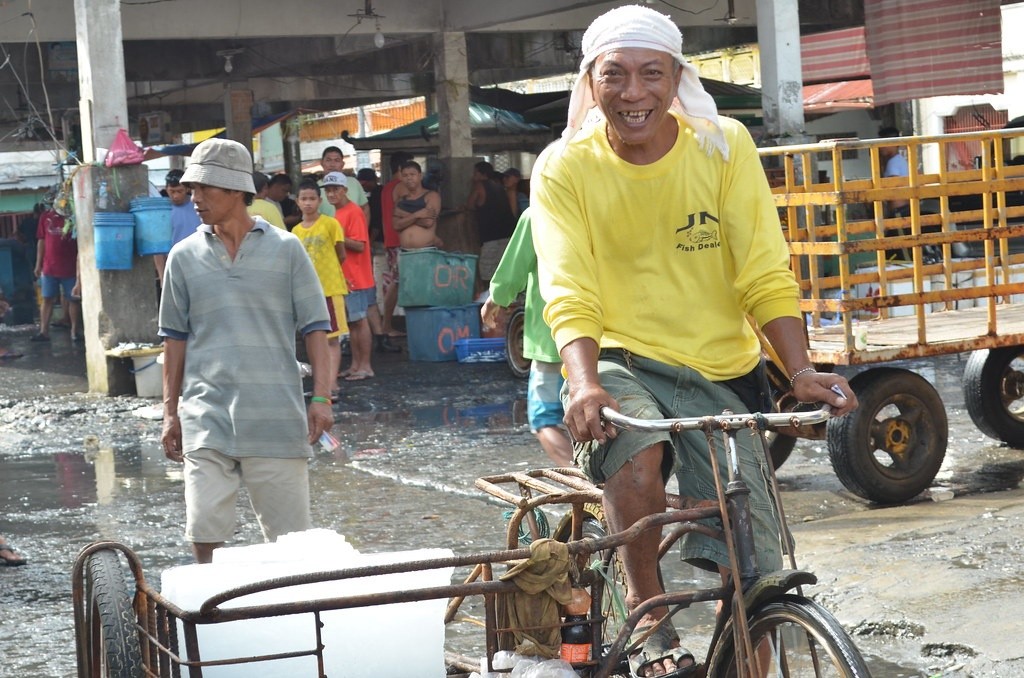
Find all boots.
<box><xmin>374</xmin><ymin>333</ymin><xmax>402</xmax><ymax>353</ymax></box>
<box><xmin>341</xmin><ymin>340</ymin><xmax>352</xmax><ymax>355</ymax></box>
<box><xmin>337</xmin><ymin>369</ymin><xmax>352</xmax><ymax>379</ymax></box>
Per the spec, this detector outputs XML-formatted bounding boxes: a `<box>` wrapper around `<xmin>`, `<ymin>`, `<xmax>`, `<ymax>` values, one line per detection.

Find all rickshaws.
<box><xmin>71</xmin><ymin>403</ymin><xmax>873</xmax><ymax>677</ymax></box>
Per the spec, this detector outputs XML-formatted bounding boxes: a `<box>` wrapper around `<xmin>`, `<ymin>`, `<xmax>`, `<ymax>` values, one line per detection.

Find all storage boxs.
<box><xmin>405</xmin><ymin>303</ymin><xmax>481</xmax><ymax>361</ymax></box>
<box><xmin>397</xmin><ymin>249</ymin><xmax>482</xmax><ymax>307</ymax></box>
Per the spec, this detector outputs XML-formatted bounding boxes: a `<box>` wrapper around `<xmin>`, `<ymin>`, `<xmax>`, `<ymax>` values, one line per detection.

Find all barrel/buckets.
<box><xmin>129</xmin><ymin>197</ymin><xmax>172</xmax><ymax>255</ymax></box>
<box><xmin>128</xmin><ymin>354</ymin><xmax>164</xmax><ymax>397</ymax></box>
<box><xmin>92</xmin><ymin>212</ymin><xmax>134</xmax><ymax>269</ymax></box>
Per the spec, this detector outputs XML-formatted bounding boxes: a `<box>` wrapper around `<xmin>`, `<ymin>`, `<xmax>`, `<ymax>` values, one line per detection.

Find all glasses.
<box><xmin>165</xmin><ymin>175</ymin><xmax>180</xmax><ymax>182</ymax></box>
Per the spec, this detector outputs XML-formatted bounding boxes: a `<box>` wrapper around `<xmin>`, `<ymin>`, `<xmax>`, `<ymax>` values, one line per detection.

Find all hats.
<box><xmin>318</xmin><ymin>171</ymin><xmax>348</xmax><ymax>188</ymax></box>
<box><xmin>179</xmin><ymin>138</ymin><xmax>257</xmax><ymax>194</ymax></box>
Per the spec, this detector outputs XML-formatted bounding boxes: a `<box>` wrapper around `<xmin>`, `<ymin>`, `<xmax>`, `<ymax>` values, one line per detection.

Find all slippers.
<box><xmin>70</xmin><ymin>334</ymin><xmax>80</xmax><ymax>342</ymax></box>
<box><xmin>29</xmin><ymin>333</ymin><xmax>50</xmax><ymax>341</ymax></box>
<box><xmin>624</xmin><ymin>621</ymin><xmax>695</xmax><ymax>678</ymax></box>
<box><xmin>344</xmin><ymin>371</ymin><xmax>374</xmax><ymax>381</ymax></box>
<box><xmin>0</xmin><ymin>349</ymin><xmax>23</xmax><ymax>358</ymax></box>
<box><xmin>0</xmin><ymin>541</ymin><xmax>26</xmax><ymax>565</ymax></box>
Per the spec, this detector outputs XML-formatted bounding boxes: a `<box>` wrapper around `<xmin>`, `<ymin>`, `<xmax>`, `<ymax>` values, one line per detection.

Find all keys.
<box><xmin>623</xmin><ymin>352</ymin><xmax>632</xmax><ymax>370</ymax></box>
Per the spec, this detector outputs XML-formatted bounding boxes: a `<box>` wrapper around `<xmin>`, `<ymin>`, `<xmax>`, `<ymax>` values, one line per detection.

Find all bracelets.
<box><xmin>790</xmin><ymin>368</ymin><xmax>816</xmax><ymax>387</ymax></box>
<box><xmin>312</xmin><ymin>396</ymin><xmax>331</xmax><ymax>406</ymax></box>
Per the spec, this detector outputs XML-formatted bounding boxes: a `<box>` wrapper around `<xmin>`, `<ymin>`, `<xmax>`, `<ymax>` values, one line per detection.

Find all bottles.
<box><xmin>558</xmin><ymin>608</ymin><xmax>592</xmax><ymax>670</ymax></box>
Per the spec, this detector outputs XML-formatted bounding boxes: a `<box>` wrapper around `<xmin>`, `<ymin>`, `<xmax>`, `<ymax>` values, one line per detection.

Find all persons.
<box><xmin>18</xmin><ymin>203</ymin><xmax>71</xmax><ymax>328</ymax></box>
<box><xmin>318</xmin><ymin>146</ymin><xmax>402</xmax><ymax>356</ymax></box>
<box><xmin>358</xmin><ymin>151</ymin><xmax>444</xmax><ymax>337</ymax></box>
<box><xmin>481</xmin><ymin>208</ymin><xmax>575</xmax><ymax>467</ymax></box>
<box><xmin>159</xmin><ymin>137</ymin><xmax>335</xmax><ymax>562</ymax></box>
<box><xmin>530</xmin><ymin>4</ymin><xmax>859</xmax><ymax>678</ymax></box>
<box><xmin>30</xmin><ymin>210</ymin><xmax>80</xmax><ymax>342</ymax></box>
<box><xmin>71</xmin><ymin>254</ymin><xmax>80</xmax><ymax>298</ymax></box>
<box><xmin>154</xmin><ymin>168</ymin><xmax>201</xmax><ymax>288</ymax></box>
<box><xmin>246</xmin><ymin>171</ymin><xmax>319</xmax><ymax>231</ymax></box>
<box><xmin>0</xmin><ymin>347</ymin><xmax>23</xmax><ymax>358</ymax></box>
<box><xmin>877</xmin><ymin>127</ymin><xmax>911</xmax><ymax>260</ymax></box>
<box><xmin>291</xmin><ymin>178</ymin><xmax>350</xmax><ymax>400</ymax></box>
<box><xmin>319</xmin><ymin>171</ymin><xmax>375</xmax><ymax>380</ymax></box>
<box><xmin>465</xmin><ymin>161</ymin><xmax>529</xmax><ymax>290</ymax></box>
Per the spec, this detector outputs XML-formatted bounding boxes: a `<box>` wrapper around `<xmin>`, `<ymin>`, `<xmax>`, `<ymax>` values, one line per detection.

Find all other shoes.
<box><xmin>331</xmin><ymin>387</ymin><xmax>341</xmax><ymax>402</ymax></box>
<box><xmin>304</xmin><ymin>389</ymin><xmax>314</xmax><ymax>397</ymax></box>
